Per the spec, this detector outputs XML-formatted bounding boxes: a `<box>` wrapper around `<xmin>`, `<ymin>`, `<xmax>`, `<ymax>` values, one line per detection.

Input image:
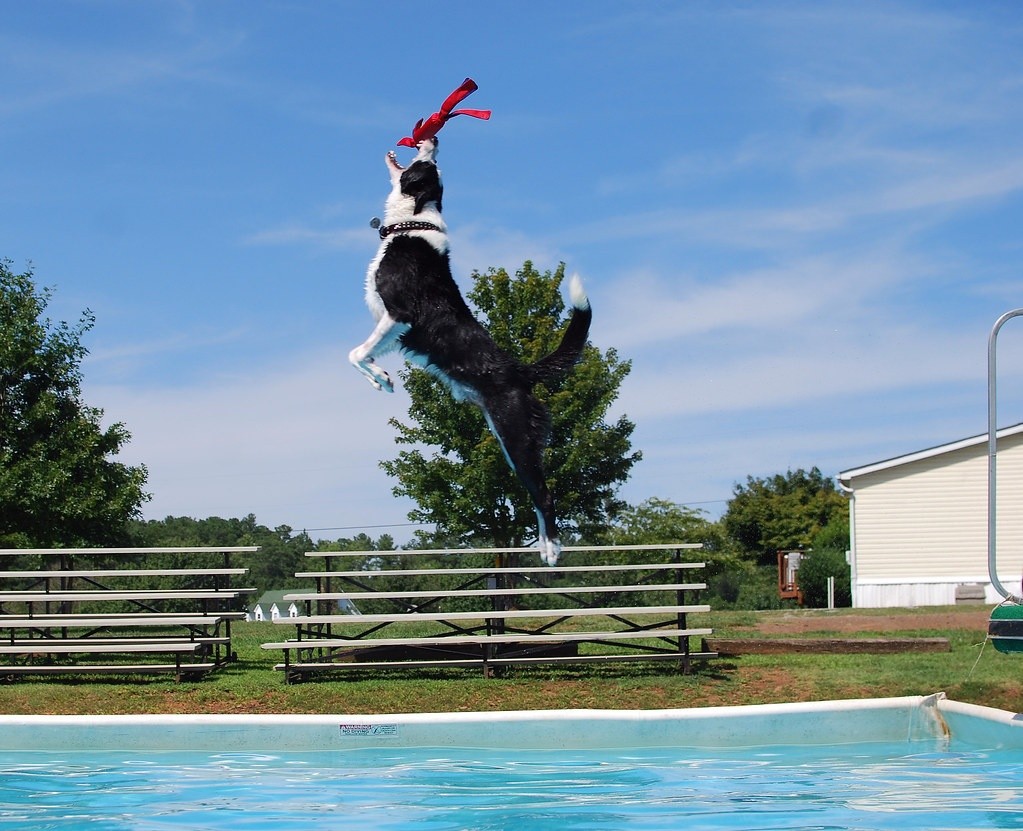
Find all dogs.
<box><xmin>346</xmin><ymin>132</ymin><xmax>590</xmax><ymax>568</ymax></box>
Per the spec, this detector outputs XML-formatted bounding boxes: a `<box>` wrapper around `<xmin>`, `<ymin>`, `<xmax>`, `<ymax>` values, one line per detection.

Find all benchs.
<box><xmin>0</xmin><ymin>543</ymin><xmax>719</xmax><ymax>685</ymax></box>
